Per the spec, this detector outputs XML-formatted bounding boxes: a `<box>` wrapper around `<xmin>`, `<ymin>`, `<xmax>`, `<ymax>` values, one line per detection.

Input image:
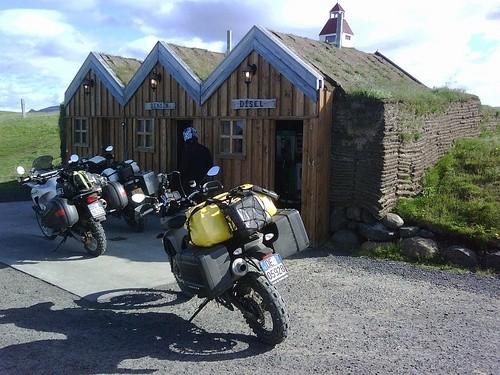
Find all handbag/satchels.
<box><xmin>184</xmin><ymin>184</ymin><xmax>277</xmax><ymax>247</ymax></box>
<box><xmin>71</xmin><ymin>170</ymin><xmax>93</xmax><ymax>193</ymax></box>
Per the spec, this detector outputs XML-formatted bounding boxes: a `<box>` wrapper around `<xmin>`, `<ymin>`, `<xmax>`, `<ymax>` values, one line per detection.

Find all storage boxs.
<box><xmin>270</xmin><ymin>208</ymin><xmax>310</xmax><ymax>259</ymax></box>
<box><xmin>175</xmin><ymin>244</ymin><xmax>233</xmax><ymax>298</ymax></box>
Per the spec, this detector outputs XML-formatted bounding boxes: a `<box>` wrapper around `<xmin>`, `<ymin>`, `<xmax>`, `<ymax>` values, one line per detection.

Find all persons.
<box><xmin>167</xmin><ymin>125</ymin><xmax>213</xmax><ymax>201</ymax></box>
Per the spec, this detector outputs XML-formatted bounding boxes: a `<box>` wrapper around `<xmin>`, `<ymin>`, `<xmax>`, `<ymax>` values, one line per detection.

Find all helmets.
<box><xmin>183</xmin><ymin>127</ymin><xmax>198</xmax><ymax>141</ymax></box>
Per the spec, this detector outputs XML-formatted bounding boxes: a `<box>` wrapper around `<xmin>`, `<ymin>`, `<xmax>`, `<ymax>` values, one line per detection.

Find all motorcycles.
<box><xmin>132</xmin><ymin>165</ymin><xmax>310</xmax><ymax>345</ymax></box>
<box><xmin>11</xmin><ymin>155</ymin><xmax>128</xmax><ymax>258</ymax></box>
<box><xmin>69</xmin><ymin>145</ymin><xmax>156</xmax><ymax>233</ymax></box>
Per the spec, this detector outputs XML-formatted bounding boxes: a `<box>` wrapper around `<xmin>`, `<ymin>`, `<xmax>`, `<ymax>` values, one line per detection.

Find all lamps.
<box><xmin>240</xmin><ymin>64</ymin><xmax>256</xmax><ymax>87</ymax></box>
<box><xmin>148</xmin><ymin>73</ymin><xmax>161</xmax><ymax>91</ymax></box>
<box><xmin>83</xmin><ymin>79</ymin><xmax>93</xmax><ymax>96</ymax></box>
